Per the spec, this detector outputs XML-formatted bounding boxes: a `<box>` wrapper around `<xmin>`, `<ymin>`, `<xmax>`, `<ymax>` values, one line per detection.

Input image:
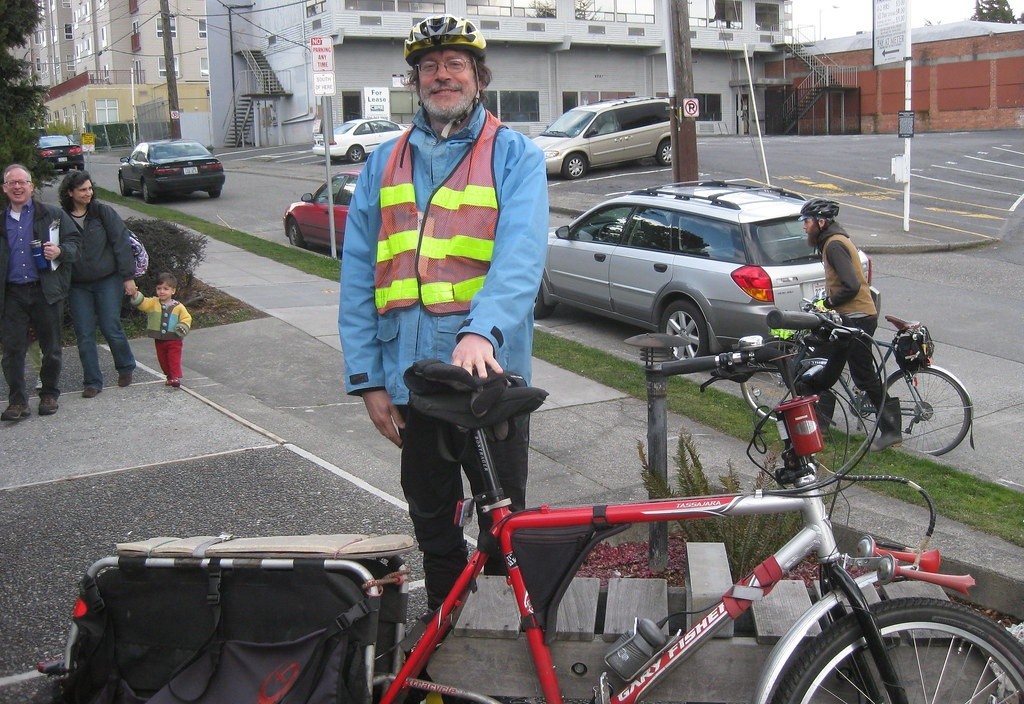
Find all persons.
<box><xmin>59</xmin><ymin>169</ymin><xmax>136</xmax><ymax>398</ymax></box>
<box><xmin>338</xmin><ymin>13</ymin><xmax>549</xmax><ymax>613</ymax></box>
<box><xmin>0</xmin><ymin>164</ymin><xmax>83</xmax><ymax>420</ymax></box>
<box><xmin>797</xmin><ymin>197</ymin><xmax>903</xmax><ymax>453</ymax></box>
<box><xmin>126</xmin><ymin>272</ymin><xmax>192</xmax><ymax>388</ymax></box>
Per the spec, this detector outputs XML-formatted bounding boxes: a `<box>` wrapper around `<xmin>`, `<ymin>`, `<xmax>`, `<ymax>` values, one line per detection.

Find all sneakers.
<box><xmin>38</xmin><ymin>398</ymin><xmax>58</xmax><ymax>415</ymax></box>
<box><xmin>1</xmin><ymin>403</ymin><xmax>31</xmax><ymax>421</ymax></box>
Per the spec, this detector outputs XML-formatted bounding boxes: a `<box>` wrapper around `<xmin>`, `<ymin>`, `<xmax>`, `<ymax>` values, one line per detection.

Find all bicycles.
<box><xmin>730</xmin><ymin>287</ymin><xmax>976</xmax><ymax>457</ymax></box>
<box><xmin>37</xmin><ymin>305</ymin><xmax>1023</xmax><ymax>703</ymax></box>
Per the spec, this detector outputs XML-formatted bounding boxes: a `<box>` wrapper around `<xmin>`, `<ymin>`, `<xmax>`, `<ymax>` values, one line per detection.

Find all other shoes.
<box><xmin>118</xmin><ymin>372</ymin><xmax>132</xmax><ymax>387</ymax></box>
<box><xmin>169</xmin><ymin>376</ymin><xmax>180</xmax><ymax>389</ymax></box>
<box><xmin>82</xmin><ymin>387</ymin><xmax>99</xmax><ymax>398</ymax></box>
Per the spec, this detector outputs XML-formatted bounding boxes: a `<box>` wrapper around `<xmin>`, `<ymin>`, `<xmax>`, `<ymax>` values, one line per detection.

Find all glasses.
<box><xmin>5</xmin><ymin>179</ymin><xmax>30</xmax><ymax>186</ymax></box>
<box><xmin>414</xmin><ymin>57</ymin><xmax>469</xmax><ymax>74</ymax></box>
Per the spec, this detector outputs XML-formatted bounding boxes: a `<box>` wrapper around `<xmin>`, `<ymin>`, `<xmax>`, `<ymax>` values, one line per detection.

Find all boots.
<box><xmin>869</xmin><ymin>399</ymin><xmax>903</xmax><ymax>451</ymax></box>
<box><xmin>811</xmin><ymin>394</ymin><xmax>836</xmax><ymax>435</ymax></box>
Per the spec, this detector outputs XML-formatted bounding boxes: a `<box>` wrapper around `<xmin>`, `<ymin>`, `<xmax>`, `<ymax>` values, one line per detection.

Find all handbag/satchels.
<box><xmin>98</xmin><ymin>202</ymin><xmax>149</xmax><ymax>278</ymax></box>
<box><xmin>896</xmin><ymin>327</ymin><xmax>934</xmax><ymax>372</ymax></box>
<box><xmin>67</xmin><ymin>556</ymin><xmax>408</xmax><ymax>704</ymax></box>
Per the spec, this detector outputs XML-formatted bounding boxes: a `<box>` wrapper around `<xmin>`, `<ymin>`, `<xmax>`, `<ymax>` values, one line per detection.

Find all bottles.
<box><xmin>605</xmin><ymin>620</ymin><xmax>666</xmax><ymax>680</ymax></box>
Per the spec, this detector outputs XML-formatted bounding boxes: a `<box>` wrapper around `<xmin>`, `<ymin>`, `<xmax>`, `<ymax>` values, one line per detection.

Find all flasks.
<box><xmin>29</xmin><ymin>238</ymin><xmax>49</xmax><ymax>270</ymax></box>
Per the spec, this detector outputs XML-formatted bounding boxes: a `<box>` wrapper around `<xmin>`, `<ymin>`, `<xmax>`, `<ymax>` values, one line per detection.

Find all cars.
<box><xmin>282</xmin><ymin>171</ymin><xmax>360</xmax><ymax>253</ymax></box>
<box><xmin>312</xmin><ymin>118</ymin><xmax>410</xmax><ymax>164</ymax></box>
<box><xmin>37</xmin><ymin>135</ymin><xmax>86</xmax><ymax>172</ymax></box>
<box><xmin>116</xmin><ymin>138</ymin><xmax>225</xmax><ymax>203</ymax></box>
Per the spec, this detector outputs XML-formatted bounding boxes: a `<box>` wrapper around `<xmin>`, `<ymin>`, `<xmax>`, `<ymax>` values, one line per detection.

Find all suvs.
<box><xmin>531</xmin><ymin>96</ymin><xmax>675</xmax><ymax>182</ymax></box>
<box><xmin>531</xmin><ymin>179</ymin><xmax>882</xmax><ymax>360</ymax></box>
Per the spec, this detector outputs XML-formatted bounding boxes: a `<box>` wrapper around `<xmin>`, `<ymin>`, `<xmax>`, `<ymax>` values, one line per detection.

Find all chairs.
<box><xmin>598</xmin><ymin>114</ymin><xmax>615</xmax><ymax>135</ymax></box>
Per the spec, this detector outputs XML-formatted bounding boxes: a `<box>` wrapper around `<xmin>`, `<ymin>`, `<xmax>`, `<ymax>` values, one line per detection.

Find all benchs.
<box><xmin>427</xmin><ymin>543</ymin><xmax>1020</xmax><ymax>704</ymax></box>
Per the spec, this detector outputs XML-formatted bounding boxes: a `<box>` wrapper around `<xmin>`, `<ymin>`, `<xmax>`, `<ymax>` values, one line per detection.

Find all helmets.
<box><xmin>404</xmin><ymin>13</ymin><xmax>487</xmax><ymax>68</ymax></box>
<box><xmin>798</xmin><ymin>198</ymin><xmax>840</xmax><ymax>223</ymax></box>
